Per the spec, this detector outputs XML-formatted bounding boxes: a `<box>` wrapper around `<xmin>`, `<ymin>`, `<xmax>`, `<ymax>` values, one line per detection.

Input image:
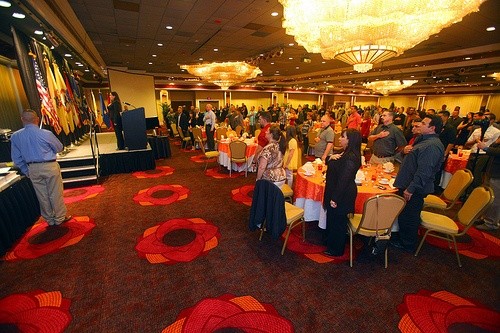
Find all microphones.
<box><xmin>124</xmin><ymin>102</ymin><xmax>136</xmax><ymax>110</ymax></box>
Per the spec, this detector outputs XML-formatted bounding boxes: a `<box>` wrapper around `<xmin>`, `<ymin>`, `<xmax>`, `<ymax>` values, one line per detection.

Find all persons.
<box><xmin>11</xmin><ymin>109</ymin><xmax>72</xmax><ymax>227</ymax></box>
<box><xmin>389</xmin><ymin>114</ymin><xmax>445</xmax><ymax>250</ymax></box>
<box><xmin>323</xmin><ymin>129</ymin><xmax>362</xmax><ymax>256</ymax></box>
<box><xmin>107</xmin><ymin>91</ymin><xmax>124</xmax><ymax>150</ymax></box>
<box><xmin>166</xmin><ymin>102</ymin><xmax>500</xmax><ymax>230</ymax></box>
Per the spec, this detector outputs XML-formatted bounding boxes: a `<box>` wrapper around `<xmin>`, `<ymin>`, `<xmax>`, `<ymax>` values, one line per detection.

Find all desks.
<box><xmin>294</xmin><ymin>162</ymin><xmax>399</xmax><ymax>233</ymax></box>
<box><xmin>218</xmin><ymin>137</ymin><xmax>257</xmax><ymax>172</ymax></box>
<box><xmin>439</xmin><ymin>155</ymin><xmax>470</xmax><ymax>189</ymax></box>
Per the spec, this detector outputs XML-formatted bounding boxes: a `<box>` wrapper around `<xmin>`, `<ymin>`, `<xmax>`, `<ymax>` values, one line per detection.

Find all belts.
<box><xmin>374</xmin><ymin>153</ymin><xmax>391</xmax><ymax>158</ymax></box>
<box><xmin>27</xmin><ymin>159</ymin><xmax>56</xmax><ymax>163</ymax></box>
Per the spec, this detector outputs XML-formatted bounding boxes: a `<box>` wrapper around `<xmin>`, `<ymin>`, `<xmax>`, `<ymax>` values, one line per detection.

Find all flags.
<box><xmin>90</xmin><ymin>91</ymin><xmax>115</xmax><ymax>131</ymax></box>
<box><xmin>28</xmin><ymin>48</ymin><xmax>95</xmax><ymax>148</ymax></box>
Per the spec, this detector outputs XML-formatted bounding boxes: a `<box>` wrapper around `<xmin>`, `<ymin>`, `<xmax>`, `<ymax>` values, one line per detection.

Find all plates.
<box><xmin>0</xmin><ymin>167</ymin><xmax>11</xmax><ymax>174</ymax></box>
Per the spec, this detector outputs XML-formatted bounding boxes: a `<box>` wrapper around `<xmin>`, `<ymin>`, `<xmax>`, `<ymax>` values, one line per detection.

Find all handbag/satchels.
<box><xmin>355</xmin><ymin>236</ymin><xmax>388</xmax><ymax>263</ymax></box>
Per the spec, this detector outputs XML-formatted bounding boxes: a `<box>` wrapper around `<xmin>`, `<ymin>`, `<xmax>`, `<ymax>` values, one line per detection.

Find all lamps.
<box><xmin>363</xmin><ymin>80</ymin><xmax>418</xmax><ymax>96</ymax></box>
<box><xmin>180</xmin><ymin>61</ymin><xmax>262</xmax><ymax>90</ymax></box>
<box><xmin>278</xmin><ymin>0</ymin><xmax>485</xmax><ymax>73</ymax></box>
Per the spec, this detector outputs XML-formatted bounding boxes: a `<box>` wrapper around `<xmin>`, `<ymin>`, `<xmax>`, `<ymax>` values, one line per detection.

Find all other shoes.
<box><xmin>323</xmin><ymin>250</ymin><xmax>334</xmax><ymax>256</ymax></box>
<box><xmin>63</xmin><ymin>215</ymin><xmax>72</xmax><ymax>222</ymax></box>
<box><xmin>389</xmin><ymin>241</ymin><xmax>408</xmax><ymax>250</ymax></box>
<box><xmin>459</xmin><ymin>195</ymin><xmax>468</xmax><ymax>201</ymax></box>
<box><xmin>476</xmin><ymin>224</ymin><xmax>495</xmax><ymax>231</ymax></box>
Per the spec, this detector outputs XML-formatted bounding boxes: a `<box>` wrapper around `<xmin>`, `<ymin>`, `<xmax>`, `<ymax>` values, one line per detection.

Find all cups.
<box><xmin>312</xmin><ymin>162</ymin><xmax>322</xmax><ymax>170</ymax></box>
<box><xmin>221</xmin><ymin>134</ymin><xmax>246</xmax><ymax>143</ymax></box>
<box><xmin>254</xmin><ymin>138</ymin><xmax>257</xmax><ymax>143</ymax></box>
<box><xmin>459</xmin><ymin>152</ymin><xmax>463</xmax><ymax>158</ymax></box>
<box><xmin>364</xmin><ymin>161</ymin><xmax>383</xmax><ymax>187</ymax></box>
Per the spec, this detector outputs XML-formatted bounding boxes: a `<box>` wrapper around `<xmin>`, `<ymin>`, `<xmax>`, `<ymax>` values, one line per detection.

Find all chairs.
<box><xmin>347</xmin><ymin>193</ymin><xmax>407</xmax><ymax>268</ymax></box>
<box><xmin>236</xmin><ymin>126</ymin><xmax>242</xmax><ymax>137</ymax></box>
<box><xmin>333</xmin><ymin>133</ymin><xmax>342</xmax><ymax>150</ymax></box>
<box><xmin>171</xmin><ymin>124</ymin><xmax>178</xmax><ymax>140</ymax></box>
<box><xmin>198</xmin><ymin>136</ymin><xmax>221</xmax><ymax>174</ymax></box>
<box><xmin>414</xmin><ymin>185</ymin><xmax>495</xmax><ymax>267</ymax></box>
<box><xmin>229</xmin><ymin>140</ymin><xmax>248</xmax><ymax>178</ymax></box>
<box><xmin>423</xmin><ymin>169</ymin><xmax>474</xmax><ymax>211</ymax></box>
<box><xmin>307</xmin><ymin>132</ymin><xmax>317</xmax><ymax>156</ymax></box>
<box><xmin>281</xmin><ymin>184</ymin><xmax>293</xmax><ymax>204</ymax></box>
<box><xmin>192</xmin><ymin>128</ymin><xmax>206</xmax><ymax>147</ymax></box>
<box><xmin>214</xmin><ymin>127</ymin><xmax>227</xmax><ymax>151</ymax></box>
<box><xmin>178</xmin><ymin>126</ymin><xmax>191</xmax><ymax>149</ymax></box>
<box><xmin>255</xmin><ymin>179</ymin><xmax>305</xmax><ymax>255</ymax></box>
